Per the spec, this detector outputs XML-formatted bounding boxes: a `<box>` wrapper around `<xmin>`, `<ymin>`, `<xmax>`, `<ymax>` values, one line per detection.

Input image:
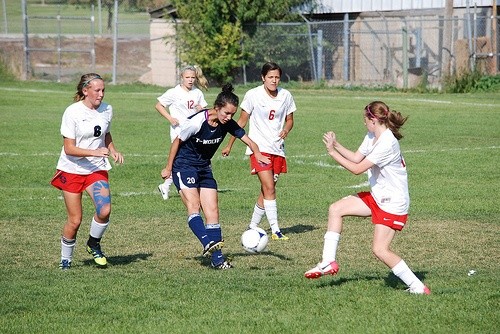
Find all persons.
<box><xmin>222</xmin><ymin>62</ymin><xmax>297</xmax><ymax>240</ymax></box>
<box><xmin>49</xmin><ymin>73</ymin><xmax>123</xmax><ymax>270</ymax></box>
<box><xmin>155</xmin><ymin>65</ymin><xmax>208</xmax><ymax>200</ymax></box>
<box><xmin>304</xmin><ymin>102</ymin><xmax>430</xmax><ymax>294</ymax></box>
<box><xmin>161</xmin><ymin>82</ymin><xmax>271</xmax><ymax>269</ymax></box>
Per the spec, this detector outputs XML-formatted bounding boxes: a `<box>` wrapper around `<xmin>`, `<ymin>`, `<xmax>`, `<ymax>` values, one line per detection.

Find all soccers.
<box><xmin>240</xmin><ymin>226</ymin><xmax>268</xmax><ymax>254</ymax></box>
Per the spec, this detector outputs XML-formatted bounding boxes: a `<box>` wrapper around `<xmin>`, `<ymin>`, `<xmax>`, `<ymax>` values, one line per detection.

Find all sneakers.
<box><xmin>59</xmin><ymin>260</ymin><xmax>70</xmax><ymax>270</ymax></box>
<box><xmin>211</xmin><ymin>260</ymin><xmax>232</xmax><ymax>270</ymax></box>
<box><xmin>304</xmin><ymin>260</ymin><xmax>338</xmax><ymax>279</ymax></box>
<box><xmin>273</xmin><ymin>232</ymin><xmax>289</xmax><ymax>241</ymax></box>
<box><xmin>203</xmin><ymin>241</ymin><xmax>225</xmax><ymax>258</ymax></box>
<box><xmin>158</xmin><ymin>183</ymin><xmax>168</xmax><ymax>200</ymax></box>
<box><xmin>85</xmin><ymin>241</ymin><xmax>106</xmax><ymax>266</ymax></box>
<box><xmin>403</xmin><ymin>285</ymin><xmax>432</xmax><ymax>294</ymax></box>
